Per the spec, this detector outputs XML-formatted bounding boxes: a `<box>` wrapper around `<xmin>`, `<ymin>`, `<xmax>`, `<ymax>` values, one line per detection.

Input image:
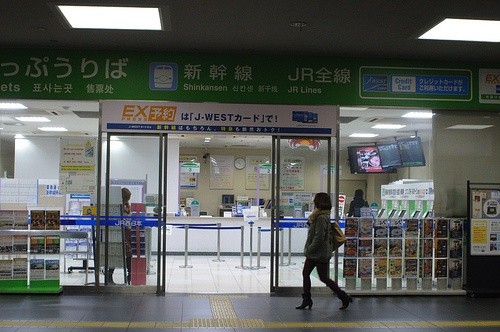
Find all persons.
<box><xmin>108</xmin><ymin>188</ymin><xmax>132</xmax><ymax>285</ymax></box>
<box><xmin>348</xmin><ymin>189</ymin><xmax>369</xmax><ymax>217</ymax></box>
<box><xmin>296</xmin><ymin>193</ymin><xmax>353</xmax><ymax>310</ymax></box>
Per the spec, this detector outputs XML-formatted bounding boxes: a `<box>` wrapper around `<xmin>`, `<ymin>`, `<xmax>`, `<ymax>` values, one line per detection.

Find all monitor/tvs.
<box><xmin>221</xmin><ymin>194</ymin><xmax>235</xmax><ymax>208</ymax></box>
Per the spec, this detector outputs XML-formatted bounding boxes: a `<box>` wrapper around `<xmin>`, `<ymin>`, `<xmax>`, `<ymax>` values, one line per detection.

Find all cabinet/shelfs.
<box><xmin>0</xmin><ymin>207</ymin><xmax>62</xmax><ymax>292</ymax></box>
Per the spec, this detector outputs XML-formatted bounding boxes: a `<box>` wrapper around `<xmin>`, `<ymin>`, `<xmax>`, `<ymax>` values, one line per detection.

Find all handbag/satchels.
<box><xmin>330</xmin><ymin>222</ymin><xmax>346</xmax><ymax>250</ymax></box>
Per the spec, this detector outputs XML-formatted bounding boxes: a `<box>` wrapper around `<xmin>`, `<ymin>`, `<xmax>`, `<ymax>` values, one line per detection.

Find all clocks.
<box><xmin>234</xmin><ymin>158</ymin><xmax>246</xmax><ymax>170</ymax></box>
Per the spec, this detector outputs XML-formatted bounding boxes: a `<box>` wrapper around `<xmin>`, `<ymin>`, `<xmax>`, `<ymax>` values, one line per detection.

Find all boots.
<box><xmin>295</xmin><ymin>293</ymin><xmax>312</xmax><ymax>309</ymax></box>
<box><xmin>338</xmin><ymin>292</ymin><xmax>352</xmax><ymax>310</ymax></box>
